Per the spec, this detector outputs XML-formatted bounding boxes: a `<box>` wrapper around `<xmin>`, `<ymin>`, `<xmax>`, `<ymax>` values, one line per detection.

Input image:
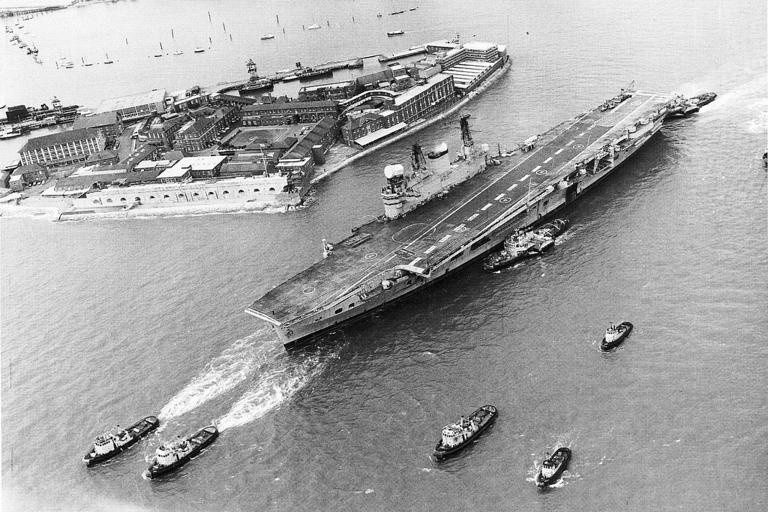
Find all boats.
<box><xmin>81</xmin><ymin>415</ymin><xmax>158</xmax><ymax>467</ymax></box>
<box><xmin>536</xmin><ymin>446</ymin><xmax>571</xmax><ymax>491</ymax></box>
<box><xmin>430</xmin><ymin>404</ymin><xmax>498</xmax><ymax>461</ymax></box>
<box><xmin>600</xmin><ymin>321</ymin><xmax>633</xmax><ymax>350</ymax></box>
<box><xmin>244</xmin><ymin>80</ymin><xmax>691</xmax><ymax>357</ymax></box>
<box><xmin>145</xmin><ymin>425</ymin><xmax>218</xmax><ymax>479</ymax></box>
<box><xmin>667</xmin><ymin>92</ymin><xmax>717</xmax><ymax>115</ymax></box>
<box><xmin>482</xmin><ymin>215</ymin><xmax>570</xmax><ymax>274</ymax></box>
